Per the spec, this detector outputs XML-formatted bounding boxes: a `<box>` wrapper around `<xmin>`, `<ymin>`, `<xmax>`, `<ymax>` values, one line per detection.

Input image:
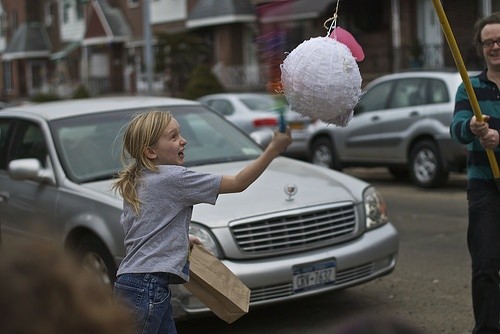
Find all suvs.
<box><xmin>304</xmin><ymin>70</ymin><xmax>482</xmax><ymax>190</ymax></box>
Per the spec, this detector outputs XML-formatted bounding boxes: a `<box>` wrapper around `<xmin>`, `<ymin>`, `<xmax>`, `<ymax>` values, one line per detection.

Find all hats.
<box><xmin>331</xmin><ymin>27</ymin><xmax>365</xmax><ymax>62</ymax></box>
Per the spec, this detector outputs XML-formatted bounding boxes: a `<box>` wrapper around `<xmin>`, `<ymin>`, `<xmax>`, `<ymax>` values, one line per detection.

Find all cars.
<box><xmin>0</xmin><ymin>94</ymin><xmax>401</xmax><ymax>323</ymax></box>
<box><xmin>184</xmin><ymin>92</ymin><xmax>316</xmax><ymax>156</ymax></box>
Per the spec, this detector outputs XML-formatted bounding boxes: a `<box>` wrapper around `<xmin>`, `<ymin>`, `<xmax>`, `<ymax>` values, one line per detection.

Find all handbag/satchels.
<box><xmin>184</xmin><ymin>244</ymin><xmax>251</xmax><ymax>324</ymax></box>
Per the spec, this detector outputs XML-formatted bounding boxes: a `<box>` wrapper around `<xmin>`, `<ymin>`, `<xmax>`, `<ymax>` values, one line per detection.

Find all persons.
<box><xmin>450</xmin><ymin>12</ymin><xmax>500</xmax><ymax>334</ymax></box>
<box><xmin>114</xmin><ymin>111</ymin><xmax>293</xmax><ymax>334</ymax></box>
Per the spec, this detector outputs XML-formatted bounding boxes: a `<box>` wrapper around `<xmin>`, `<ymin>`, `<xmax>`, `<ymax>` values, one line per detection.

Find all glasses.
<box><xmin>480</xmin><ymin>39</ymin><xmax>496</xmax><ymax>48</ymax></box>
